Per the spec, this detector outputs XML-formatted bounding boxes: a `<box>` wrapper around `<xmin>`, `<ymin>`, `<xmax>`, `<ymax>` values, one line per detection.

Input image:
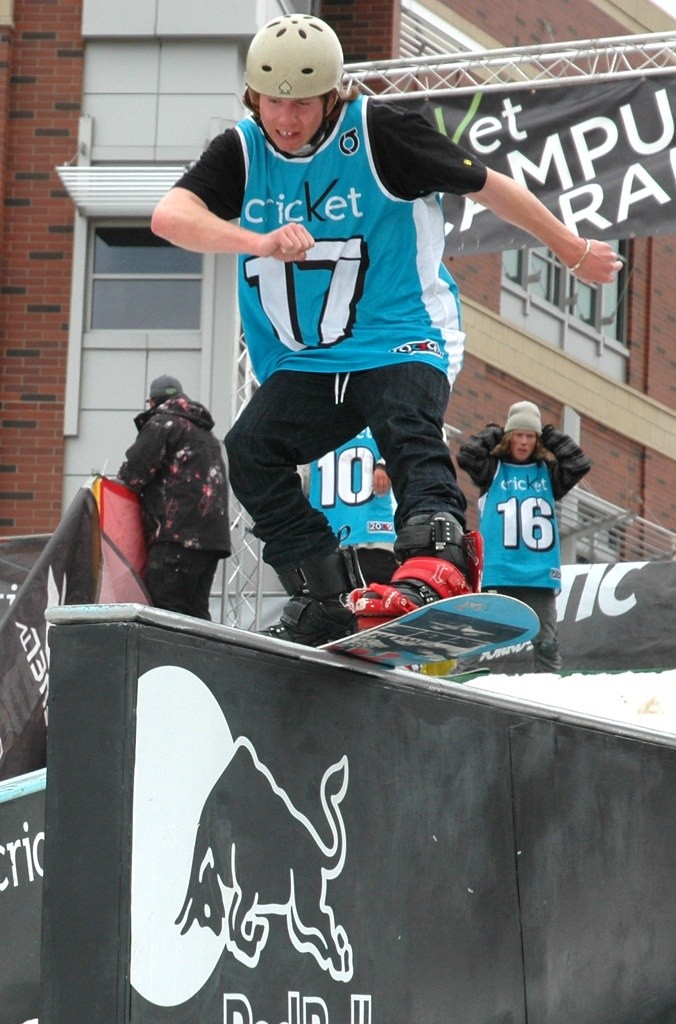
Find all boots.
<box><xmin>251</xmin><ymin>524</ymin><xmax>359</xmax><ymax>649</ymax></box>
<box><xmin>356</xmin><ymin>510</ymin><xmax>472</xmax><ymax>615</ymax></box>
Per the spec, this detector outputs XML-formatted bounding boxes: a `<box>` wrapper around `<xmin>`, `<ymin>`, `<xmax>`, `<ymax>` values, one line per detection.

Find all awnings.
<box><xmin>56</xmin><ymin>164</ymin><xmax>192</xmax><ymax>220</ymax></box>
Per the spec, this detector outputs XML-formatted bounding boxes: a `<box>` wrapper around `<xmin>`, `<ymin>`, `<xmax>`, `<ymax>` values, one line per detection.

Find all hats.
<box><xmin>145</xmin><ymin>374</ymin><xmax>184</xmax><ymax>401</ymax></box>
<box><xmin>504</xmin><ymin>401</ymin><xmax>542</xmax><ymax>435</ymax></box>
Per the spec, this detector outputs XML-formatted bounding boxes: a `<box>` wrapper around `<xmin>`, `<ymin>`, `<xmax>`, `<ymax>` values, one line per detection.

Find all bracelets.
<box><xmin>567</xmin><ymin>238</ymin><xmax>591</xmax><ymax>272</ymax></box>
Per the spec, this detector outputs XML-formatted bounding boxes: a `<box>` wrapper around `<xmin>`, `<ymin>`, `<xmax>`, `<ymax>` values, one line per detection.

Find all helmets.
<box><xmin>246</xmin><ymin>13</ymin><xmax>343</xmax><ymax>98</ymax></box>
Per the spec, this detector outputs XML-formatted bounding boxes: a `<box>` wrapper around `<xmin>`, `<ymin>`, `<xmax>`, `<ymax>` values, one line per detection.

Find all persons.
<box><xmin>309</xmin><ymin>427</ymin><xmax>397</xmax><ymax>588</ymax></box>
<box><xmin>118</xmin><ymin>375</ymin><xmax>232</xmax><ymax>622</ymax></box>
<box><xmin>151</xmin><ymin>14</ymin><xmax>624</xmax><ymax>647</ymax></box>
<box><xmin>455</xmin><ymin>402</ymin><xmax>591</xmax><ymax>673</ymax></box>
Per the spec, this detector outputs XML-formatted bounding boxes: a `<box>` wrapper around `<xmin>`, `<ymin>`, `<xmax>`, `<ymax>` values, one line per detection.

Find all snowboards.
<box><xmin>316</xmin><ymin>591</ymin><xmax>541</xmax><ymax>667</ymax></box>
